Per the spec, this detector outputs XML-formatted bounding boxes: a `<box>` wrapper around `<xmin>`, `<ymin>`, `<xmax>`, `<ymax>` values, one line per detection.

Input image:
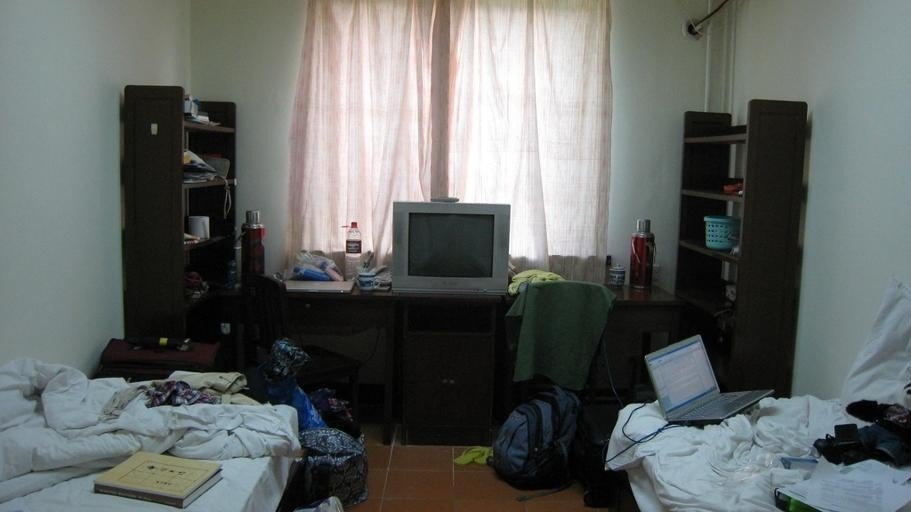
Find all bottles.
<box><xmin>345</xmin><ymin>223</ymin><xmax>362</xmax><ymax>280</ymax></box>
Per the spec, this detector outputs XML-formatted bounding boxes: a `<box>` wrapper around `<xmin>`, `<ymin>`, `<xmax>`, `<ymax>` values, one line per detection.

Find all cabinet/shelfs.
<box><xmin>277</xmin><ymin>277</ymin><xmax>675</xmax><ymax>444</ymax></box>
<box><xmin>677</xmin><ymin>100</ymin><xmax>807</xmax><ymax>394</ymax></box>
<box><xmin>122</xmin><ymin>84</ymin><xmax>236</xmax><ymax>341</ymax></box>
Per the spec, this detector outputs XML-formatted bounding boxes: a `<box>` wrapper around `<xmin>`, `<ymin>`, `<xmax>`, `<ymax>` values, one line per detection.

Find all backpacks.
<box><xmin>487</xmin><ymin>383</ymin><xmax>583</xmax><ymax>503</ymax></box>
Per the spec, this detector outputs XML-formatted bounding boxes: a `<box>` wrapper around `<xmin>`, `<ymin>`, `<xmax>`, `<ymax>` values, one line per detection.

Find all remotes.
<box><xmin>431</xmin><ymin>196</ymin><xmax>459</xmax><ymax>203</ymax></box>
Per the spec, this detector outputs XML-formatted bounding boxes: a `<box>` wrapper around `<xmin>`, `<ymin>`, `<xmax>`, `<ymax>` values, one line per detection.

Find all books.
<box><xmin>95</xmin><ymin>452</ymin><xmax>222</xmax><ymax>507</ymax></box>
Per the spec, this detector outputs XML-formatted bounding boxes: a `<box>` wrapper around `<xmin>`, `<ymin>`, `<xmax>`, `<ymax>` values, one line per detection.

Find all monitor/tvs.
<box><xmin>392</xmin><ymin>202</ymin><xmax>511</xmax><ymax>294</ymax></box>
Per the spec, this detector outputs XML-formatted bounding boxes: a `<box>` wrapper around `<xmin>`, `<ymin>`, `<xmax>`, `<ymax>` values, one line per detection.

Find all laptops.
<box><xmin>644</xmin><ymin>336</ymin><xmax>775</xmax><ymax>424</ymax></box>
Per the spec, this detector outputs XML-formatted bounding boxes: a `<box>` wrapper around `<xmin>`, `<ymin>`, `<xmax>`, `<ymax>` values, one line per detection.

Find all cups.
<box><xmin>357</xmin><ymin>273</ymin><xmax>381</xmax><ymax>293</ymax></box>
<box><xmin>189</xmin><ymin>215</ymin><xmax>211</xmax><ymax>241</ymax></box>
<box><xmin>608</xmin><ymin>268</ymin><xmax>626</xmax><ymax>287</ymax></box>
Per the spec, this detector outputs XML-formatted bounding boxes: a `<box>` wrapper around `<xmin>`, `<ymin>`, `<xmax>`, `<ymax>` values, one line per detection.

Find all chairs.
<box><xmin>517</xmin><ymin>281</ymin><xmax>625</xmax><ymax>413</ymax></box>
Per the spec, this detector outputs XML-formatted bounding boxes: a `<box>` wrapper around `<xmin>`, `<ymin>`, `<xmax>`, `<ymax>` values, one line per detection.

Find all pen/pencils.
<box><xmin>362</xmin><ymin>251</ymin><xmax>375</xmax><ymax>269</ymax></box>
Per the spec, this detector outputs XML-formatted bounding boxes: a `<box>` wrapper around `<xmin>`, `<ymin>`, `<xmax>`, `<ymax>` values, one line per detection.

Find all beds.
<box><xmin>610</xmin><ymin>401</ymin><xmax>910</xmax><ymax>512</ymax></box>
<box><xmin>1</xmin><ymin>380</ymin><xmax>297</xmax><ymax>512</ymax></box>
